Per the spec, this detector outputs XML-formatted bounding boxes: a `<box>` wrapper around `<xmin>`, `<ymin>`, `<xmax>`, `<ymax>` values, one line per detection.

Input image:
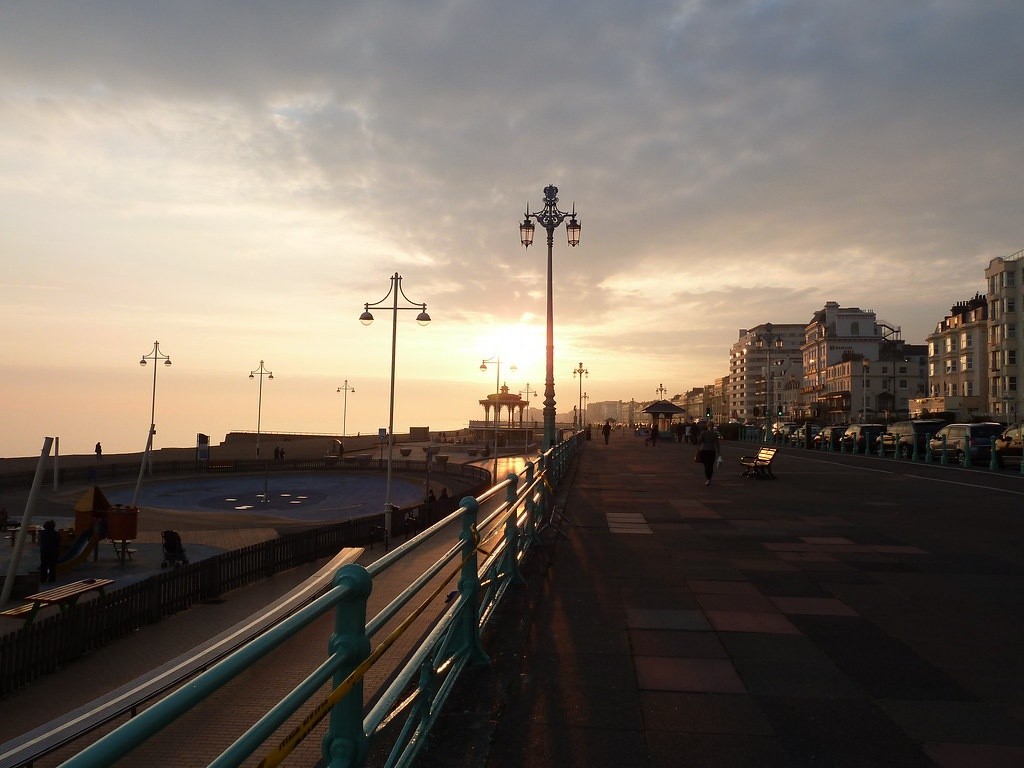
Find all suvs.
<box><xmin>773</xmin><ymin>422</ymin><xmax>887</xmax><ymax>453</ymax></box>
<box><xmin>875</xmin><ymin>419</ymin><xmax>946</xmax><ymax>459</ymax></box>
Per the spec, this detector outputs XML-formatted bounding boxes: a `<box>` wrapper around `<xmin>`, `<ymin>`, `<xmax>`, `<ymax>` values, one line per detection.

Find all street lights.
<box><xmin>480</xmin><ymin>355</ymin><xmax>517</xmax><ymax>486</ymax></box>
<box><xmin>249</xmin><ymin>360</ymin><xmax>274</xmax><ymax>433</ymax></box>
<box><xmin>140</xmin><ymin>340</ymin><xmax>172</xmax><ymax>451</ymax></box>
<box><xmin>518</xmin><ymin>184</ymin><xmax>582</xmax><ymax>453</ymax></box>
<box><xmin>359</xmin><ymin>272</ymin><xmax>431</xmax><ymax>544</ymax></box>
<box><xmin>581</xmin><ymin>392</ymin><xmax>589</xmax><ymax>426</ymax></box>
<box><xmin>573</xmin><ymin>362</ymin><xmax>589</xmax><ymax>431</ymax></box>
<box><xmin>337</xmin><ymin>379</ymin><xmax>355</xmax><ymax>436</ymax></box>
<box><xmin>656</xmin><ymin>384</ymin><xmax>667</xmax><ymax>400</ymax></box>
<box><xmin>518</xmin><ymin>383</ymin><xmax>537</xmax><ymax>454</ymax></box>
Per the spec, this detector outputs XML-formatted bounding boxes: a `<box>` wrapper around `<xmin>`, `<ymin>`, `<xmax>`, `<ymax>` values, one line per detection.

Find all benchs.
<box><xmin>739</xmin><ymin>446</ymin><xmax>778</xmax><ymax>479</ymax></box>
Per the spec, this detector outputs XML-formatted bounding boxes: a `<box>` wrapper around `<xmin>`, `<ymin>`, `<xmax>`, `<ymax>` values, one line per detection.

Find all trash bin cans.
<box><xmin>586</xmin><ymin>429</ymin><xmax>591</xmax><ymax>441</ymax></box>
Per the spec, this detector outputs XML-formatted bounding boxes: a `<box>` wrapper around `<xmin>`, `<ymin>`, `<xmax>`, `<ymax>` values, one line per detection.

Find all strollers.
<box><xmin>161</xmin><ymin>530</ymin><xmax>189</xmax><ymax>568</ymax></box>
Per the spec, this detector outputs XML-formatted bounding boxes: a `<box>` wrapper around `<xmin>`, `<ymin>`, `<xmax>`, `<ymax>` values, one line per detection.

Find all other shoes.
<box><xmin>704</xmin><ymin>480</ymin><xmax>710</xmax><ymax>488</ymax></box>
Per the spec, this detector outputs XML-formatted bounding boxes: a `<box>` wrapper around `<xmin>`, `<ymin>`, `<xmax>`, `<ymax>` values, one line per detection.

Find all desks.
<box><xmin>8</xmin><ymin>528</ymin><xmax>40</xmax><ymax>546</ymax></box>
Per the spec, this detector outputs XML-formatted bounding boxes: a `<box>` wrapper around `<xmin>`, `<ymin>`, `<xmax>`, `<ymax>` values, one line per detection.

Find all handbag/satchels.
<box><xmin>694</xmin><ymin>449</ymin><xmax>703</xmax><ymax>464</ymax></box>
<box><xmin>716</xmin><ymin>456</ymin><xmax>723</xmax><ymax>474</ymax></box>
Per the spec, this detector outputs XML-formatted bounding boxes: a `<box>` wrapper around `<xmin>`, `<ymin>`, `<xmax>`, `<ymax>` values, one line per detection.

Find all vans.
<box><xmin>995</xmin><ymin>420</ymin><xmax>1024</xmax><ymax>465</ymax></box>
<box><xmin>926</xmin><ymin>422</ymin><xmax>1004</xmax><ymax>465</ymax></box>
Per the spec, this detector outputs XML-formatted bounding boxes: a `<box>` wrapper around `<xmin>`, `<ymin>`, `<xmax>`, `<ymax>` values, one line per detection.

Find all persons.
<box><xmin>273</xmin><ymin>445</ymin><xmax>280</xmax><ymax>462</ymax></box>
<box><xmin>433</xmin><ymin>431</ymin><xmax>467</xmax><ymax>442</ymax></box>
<box><xmin>696</xmin><ymin>421</ymin><xmax>719</xmax><ymax>486</ymax></box>
<box><xmin>39</xmin><ymin>519</ymin><xmax>63</xmax><ymax>584</ymax></box>
<box><xmin>424</xmin><ymin>489</ymin><xmax>436</xmax><ymax>503</ymax></box>
<box><xmin>95</xmin><ymin>441</ymin><xmax>102</xmax><ymax>460</ymax></box>
<box><xmin>601</xmin><ymin>421</ymin><xmax>611</xmax><ymax>445</ymax></box>
<box><xmin>650</xmin><ymin>425</ymin><xmax>658</xmax><ymax>447</ymax></box>
<box><xmin>437</xmin><ymin>488</ymin><xmax>449</xmax><ymax>499</ymax></box>
<box><xmin>676</xmin><ymin>422</ymin><xmax>683</xmax><ymax>443</ymax></box>
<box><xmin>644</xmin><ymin>429</ymin><xmax>650</xmax><ymax>447</ymax></box>
<box><xmin>683</xmin><ymin>421</ymin><xmax>703</xmax><ymax>445</ymax></box>
<box><xmin>339</xmin><ymin>446</ymin><xmax>344</xmax><ymax>458</ymax></box>
<box><xmin>280</xmin><ymin>448</ymin><xmax>285</xmax><ymax>462</ymax></box>
